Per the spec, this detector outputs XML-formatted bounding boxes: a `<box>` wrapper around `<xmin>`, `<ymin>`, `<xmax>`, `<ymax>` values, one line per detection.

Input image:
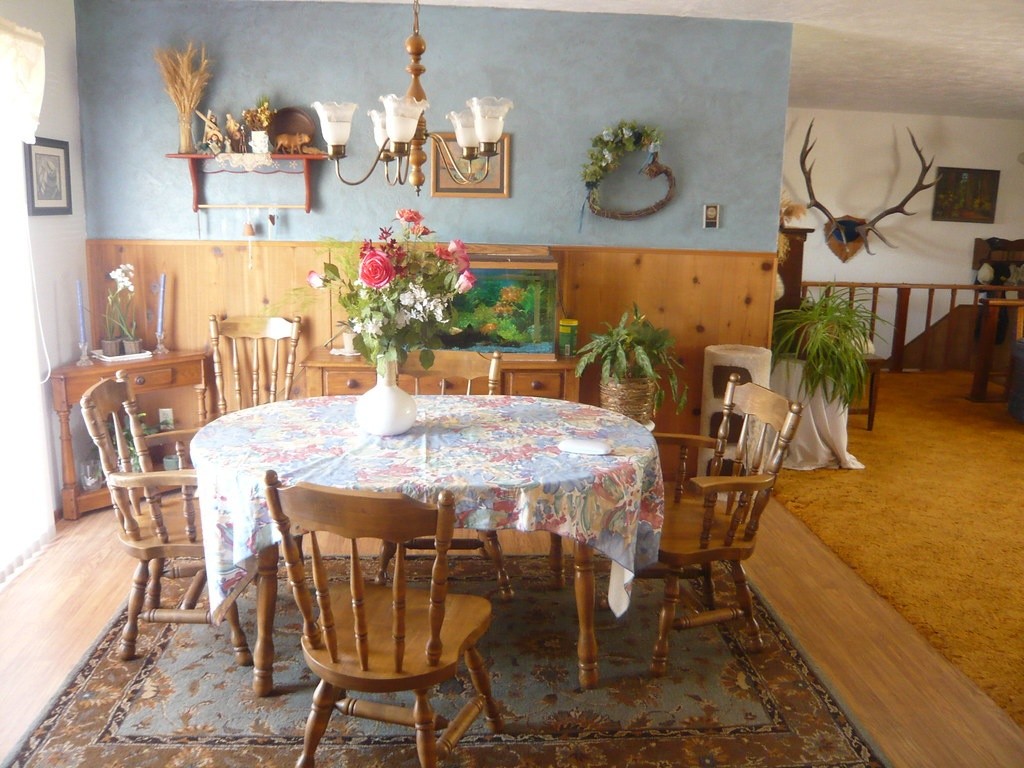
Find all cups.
<box><xmin>163</xmin><ymin>454</ymin><xmax>179</xmax><ymax>471</ymax></box>
<box><xmin>80</xmin><ymin>460</ymin><xmax>106</xmax><ymax>491</ymax></box>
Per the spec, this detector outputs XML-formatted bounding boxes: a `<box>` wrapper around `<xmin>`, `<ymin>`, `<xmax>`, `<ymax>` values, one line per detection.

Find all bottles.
<box><xmin>558</xmin><ymin>318</ymin><xmax>579</xmax><ymax>357</ymax></box>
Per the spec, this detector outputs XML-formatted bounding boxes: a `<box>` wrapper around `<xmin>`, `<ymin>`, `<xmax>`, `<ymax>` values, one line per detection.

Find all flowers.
<box><xmin>82</xmin><ymin>263</ymin><xmax>136</xmax><ymax>341</ymax></box>
<box><xmin>305</xmin><ymin>208</ymin><xmax>477</xmax><ymax>377</ymax></box>
<box><xmin>242</xmin><ymin>93</ymin><xmax>278</xmax><ymax>131</ymax></box>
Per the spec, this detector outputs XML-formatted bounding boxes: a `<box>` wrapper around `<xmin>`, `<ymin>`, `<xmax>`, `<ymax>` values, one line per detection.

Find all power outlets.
<box><xmin>159</xmin><ymin>408</ymin><xmax>174</xmax><ymax>430</ymax></box>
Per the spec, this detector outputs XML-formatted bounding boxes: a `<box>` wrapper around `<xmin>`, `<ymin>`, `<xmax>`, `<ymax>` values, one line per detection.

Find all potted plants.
<box><xmin>769</xmin><ymin>276</ymin><xmax>898</xmax><ymax>417</ymax></box>
<box><xmin>574</xmin><ymin>300</ymin><xmax>689</xmax><ymax>424</ymax></box>
<box><xmin>86</xmin><ymin>412</ymin><xmax>175</xmax><ymax>473</ymax></box>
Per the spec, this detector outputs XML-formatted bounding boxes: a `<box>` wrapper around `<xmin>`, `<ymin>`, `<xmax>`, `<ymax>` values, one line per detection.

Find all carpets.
<box><xmin>0</xmin><ymin>553</ymin><xmax>893</xmax><ymax>768</ymax></box>
<box><xmin>772</xmin><ymin>367</ymin><xmax>1023</xmax><ymax>731</ymax></box>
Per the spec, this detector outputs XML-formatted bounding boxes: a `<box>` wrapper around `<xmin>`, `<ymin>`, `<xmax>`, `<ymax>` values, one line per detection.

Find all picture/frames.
<box><xmin>932</xmin><ymin>167</ymin><xmax>1000</xmax><ymax>224</ymax></box>
<box><xmin>431</xmin><ymin>132</ymin><xmax>512</xmax><ymax>198</ymax></box>
<box><xmin>24</xmin><ymin>136</ymin><xmax>73</xmax><ymax>216</ymax></box>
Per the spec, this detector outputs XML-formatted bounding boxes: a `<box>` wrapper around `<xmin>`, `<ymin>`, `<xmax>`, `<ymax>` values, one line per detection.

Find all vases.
<box><xmin>163</xmin><ymin>454</ymin><xmax>181</xmax><ymax>470</ymax></box>
<box><xmin>248</xmin><ymin>131</ymin><xmax>269</xmax><ymax>154</ymax></box>
<box><xmin>178</xmin><ymin>112</ymin><xmax>200</xmax><ymax>155</ymax></box>
<box><xmin>356</xmin><ymin>354</ymin><xmax>417</xmax><ymax>436</ymax></box>
<box><xmin>99</xmin><ymin>338</ymin><xmax>146</xmax><ymax>357</ymax></box>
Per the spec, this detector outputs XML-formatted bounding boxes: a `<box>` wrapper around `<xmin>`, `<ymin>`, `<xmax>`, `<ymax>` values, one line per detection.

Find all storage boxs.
<box><xmin>432</xmin><ymin>254</ymin><xmax>559</xmax><ymax>362</ymax></box>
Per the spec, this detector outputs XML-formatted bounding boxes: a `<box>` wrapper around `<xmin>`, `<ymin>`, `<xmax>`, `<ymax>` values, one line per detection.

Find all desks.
<box><xmin>190</xmin><ymin>392</ymin><xmax>664</xmax><ymax>699</ymax></box>
<box><xmin>848</xmin><ymin>353</ymin><xmax>885</xmax><ymax>432</ymax></box>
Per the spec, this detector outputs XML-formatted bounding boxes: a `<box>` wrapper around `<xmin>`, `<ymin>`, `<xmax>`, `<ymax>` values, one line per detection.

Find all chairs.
<box><xmin>79</xmin><ymin>368</ymin><xmax>257</xmax><ymax>666</ymax></box>
<box><xmin>264</xmin><ymin>469</ymin><xmax>505</xmax><ymax>768</ymax></box>
<box><xmin>374</xmin><ymin>349</ymin><xmax>515</xmax><ymax>601</ymax></box>
<box><xmin>208</xmin><ymin>315</ymin><xmax>301</xmax><ymax>417</ymax></box>
<box><xmin>599</xmin><ymin>374</ymin><xmax>804</xmax><ymax>678</ymax></box>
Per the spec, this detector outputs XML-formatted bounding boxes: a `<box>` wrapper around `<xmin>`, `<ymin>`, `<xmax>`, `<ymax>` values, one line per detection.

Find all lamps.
<box><xmin>311</xmin><ymin>0</ymin><xmax>514</xmax><ymax>196</ymax></box>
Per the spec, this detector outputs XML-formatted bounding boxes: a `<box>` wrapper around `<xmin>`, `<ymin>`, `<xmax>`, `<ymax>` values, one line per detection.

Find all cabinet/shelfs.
<box><xmin>298</xmin><ymin>345</ymin><xmax>581</xmax><ymax>404</ymax></box>
<box><xmin>48</xmin><ymin>351</ymin><xmax>210</xmax><ymax>520</ymax></box>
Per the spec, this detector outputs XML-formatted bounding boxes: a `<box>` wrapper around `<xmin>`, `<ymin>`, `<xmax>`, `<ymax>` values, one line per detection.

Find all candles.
<box><xmin>76</xmin><ymin>279</ymin><xmax>86</xmax><ymax>343</ymax></box>
<box><xmin>156</xmin><ymin>273</ymin><xmax>166</xmax><ymax>337</ymax></box>
